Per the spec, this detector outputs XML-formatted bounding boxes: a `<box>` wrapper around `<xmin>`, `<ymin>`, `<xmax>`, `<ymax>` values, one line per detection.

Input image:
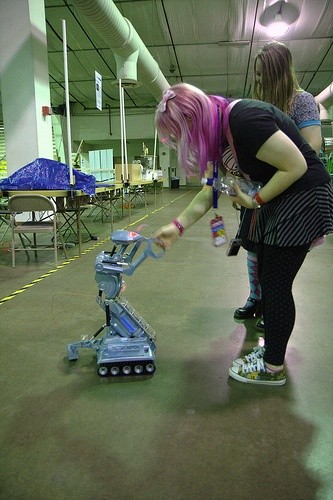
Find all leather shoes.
<box><xmin>255</xmin><ymin>318</ymin><xmax>265</xmax><ymax>332</ymax></box>
<box><xmin>234</xmin><ymin>303</ymin><xmax>263</xmax><ymax>319</ymax></box>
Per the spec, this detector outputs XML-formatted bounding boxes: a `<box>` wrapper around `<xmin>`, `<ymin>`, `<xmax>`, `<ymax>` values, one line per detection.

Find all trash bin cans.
<box><xmin>171</xmin><ymin>176</ymin><xmax>180</xmax><ymax>190</ymax></box>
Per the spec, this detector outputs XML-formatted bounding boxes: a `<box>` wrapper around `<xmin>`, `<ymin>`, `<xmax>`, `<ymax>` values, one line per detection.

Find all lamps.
<box><xmin>260</xmin><ymin>0</ymin><xmax>299</xmax><ymax>35</ymax></box>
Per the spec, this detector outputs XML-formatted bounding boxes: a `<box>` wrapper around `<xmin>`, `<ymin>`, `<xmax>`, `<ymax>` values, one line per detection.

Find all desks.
<box><xmin>4</xmin><ymin>178</ymin><xmax>164</xmax><ymax>258</ymax></box>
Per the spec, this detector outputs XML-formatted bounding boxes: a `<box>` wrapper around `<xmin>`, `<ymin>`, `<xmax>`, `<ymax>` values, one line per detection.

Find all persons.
<box><xmin>234</xmin><ymin>41</ymin><xmax>322</xmax><ymax>331</ymax></box>
<box><xmin>150</xmin><ymin>83</ymin><xmax>333</xmax><ymax>385</ymax></box>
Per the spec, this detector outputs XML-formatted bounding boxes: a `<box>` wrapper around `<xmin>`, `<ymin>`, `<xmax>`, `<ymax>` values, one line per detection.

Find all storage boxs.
<box><xmin>114</xmin><ymin>163</ymin><xmax>141</xmax><ymax>183</ymax></box>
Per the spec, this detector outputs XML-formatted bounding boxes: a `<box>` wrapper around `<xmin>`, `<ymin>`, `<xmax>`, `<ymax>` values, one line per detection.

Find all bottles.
<box><xmin>213</xmin><ymin>177</ymin><xmax>262</xmax><ymax>195</ymax></box>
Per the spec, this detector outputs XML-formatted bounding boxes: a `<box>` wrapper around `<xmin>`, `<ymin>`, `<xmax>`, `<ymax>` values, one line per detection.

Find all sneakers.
<box><xmin>228</xmin><ymin>357</ymin><xmax>287</xmax><ymax>385</ymax></box>
<box><xmin>233</xmin><ymin>346</ymin><xmax>265</xmax><ymax>367</ymax></box>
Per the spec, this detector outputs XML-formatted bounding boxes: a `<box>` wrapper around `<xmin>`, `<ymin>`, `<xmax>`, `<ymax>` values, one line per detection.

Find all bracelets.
<box><xmin>173</xmin><ymin>218</ymin><xmax>185</xmax><ymax>235</ymax></box>
<box><xmin>251</xmin><ymin>193</ymin><xmax>266</xmax><ymax>209</ymax></box>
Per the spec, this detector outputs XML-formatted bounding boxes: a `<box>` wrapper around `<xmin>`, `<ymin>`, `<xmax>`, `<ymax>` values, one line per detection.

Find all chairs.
<box><xmin>0</xmin><ymin>187</ymin><xmax>151</xmax><ymax>268</ymax></box>
